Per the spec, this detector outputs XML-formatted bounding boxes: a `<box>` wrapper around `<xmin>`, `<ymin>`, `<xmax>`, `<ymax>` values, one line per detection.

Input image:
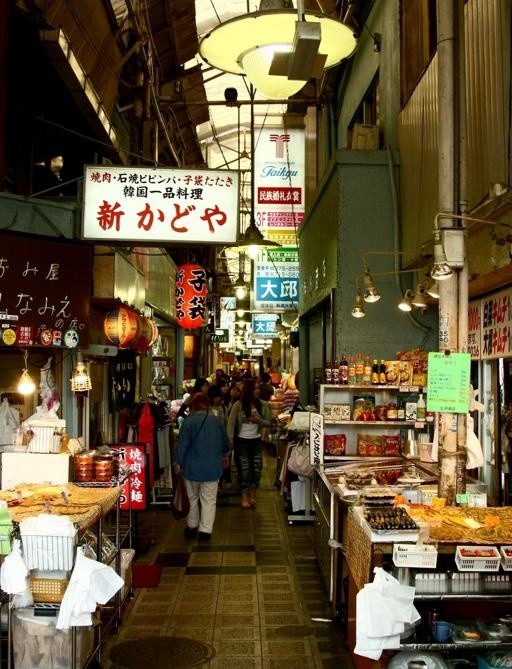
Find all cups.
<box><xmin>435</xmin><ymin>621</ymin><xmax>453</xmax><ymax>641</ymax></box>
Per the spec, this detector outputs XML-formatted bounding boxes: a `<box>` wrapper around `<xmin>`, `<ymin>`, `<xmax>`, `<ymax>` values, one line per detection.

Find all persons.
<box><xmin>228</xmin><ymin>378</ymin><xmax>271</xmax><ymax>509</ymax></box>
<box><xmin>190</xmin><ymin>360</ymin><xmax>274</xmax><ymax>406</ymax></box>
<box><xmin>175</xmin><ymin>376</ymin><xmax>209</xmax><ymax>498</ymax></box>
<box><xmin>173</xmin><ymin>394</ymin><xmax>229</xmax><ymax>543</ymax></box>
<box><xmin>208</xmin><ymin>384</ymin><xmax>232</xmax><ymax>498</ymax></box>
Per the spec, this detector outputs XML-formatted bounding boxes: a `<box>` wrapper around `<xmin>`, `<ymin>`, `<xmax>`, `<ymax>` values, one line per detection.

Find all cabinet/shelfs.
<box><xmin>319</xmin><ymin>382</ymin><xmax>440</xmax><ymax>475</ymax></box>
<box><xmin>312</xmin><ymin>465</ymin><xmax>489</xmax><ymax>603</ymax></box>
<box><xmin>0</xmin><ymin>471</ymin><xmax>135</xmax><ymax>669</ymax></box>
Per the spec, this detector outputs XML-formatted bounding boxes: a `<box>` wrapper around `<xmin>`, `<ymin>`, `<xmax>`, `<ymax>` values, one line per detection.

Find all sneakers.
<box><xmin>240</xmin><ymin>501</ymin><xmax>250</xmax><ymax>508</ymax></box>
<box><xmin>250</xmin><ymin>499</ymin><xmax>256</xmax><ymax>504</ymax></box>
<box><xmin>184</xmin><ymin>527</ymin><xmax>198</xmax><ymax>539</ymax></box>
<box><xmin>198</xmin><ymin>532</ymin><xmax>211</xmax><ymax>542</ymax></box>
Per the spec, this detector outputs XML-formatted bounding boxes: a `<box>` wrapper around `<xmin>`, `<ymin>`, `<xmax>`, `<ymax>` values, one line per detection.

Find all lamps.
<box><xmin>350</xmin><ymin>268</ymin><xmax>428</xmax><ymax>318</ymax></box>
<box><xmin>410</xmin><ymin>283</ymin><xmax>427</xmax><ymax>307</ymax></box>
<box><xmin>71</xmin><ymin>347</ymin><xmax>93</xmax><ymax>392</ymax></box>
<box><xmin>361</xmin><ymin>250</ymin><xmax>426</xmax><ymax>303</ymax></box>
<box><xmin>428</xmin><ymin>212</ymin><xmax>511</xmax><ymax>282</ymax></box>
<box><xmin>220</xmin><ymin>107</ymin><xmax>282</xmax><ymax>257</ymax></box>
<box><xmin>198</xmin><ymin>0</ymin><xmax>359</xmax><ymax>101</ymax></box>
<box><xmin>397</xmin><ymin>289</ymin><xmax>414</xmax><ymax>312</ymax></box>
<box><xmin>426</xmin><ymin>282</ymin><xmax>441</xmax><ymax>301</ymax></box>
<box><xmin>231</xmin><ymin>258</ymin><xmax>246</xmax><ymax>289</ymax></box>
<box><xmin>16</xmin><ymin>351</ymin><xmax>36</xmax><ymax>397</ymax></box>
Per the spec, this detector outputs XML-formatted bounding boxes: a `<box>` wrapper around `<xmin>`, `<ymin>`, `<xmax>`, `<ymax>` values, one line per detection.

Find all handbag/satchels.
<box><xmin>286</xmin><ymin>444</ymin><xmax>317</xmax><ymax>479</ymax></box>
<box><xmin>172</xmin><ymin>474</ymin><xmax>191</xmax><ymax>520</ymax></box>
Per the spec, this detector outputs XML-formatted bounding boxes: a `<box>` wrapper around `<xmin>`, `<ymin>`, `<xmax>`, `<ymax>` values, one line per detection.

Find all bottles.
<box><xmin>325</xmin><ymin>360</ymin><xmax>332</xmax><ymax>383</ymax></box>
<box><xmin>372</xmin><ymin>359</ymin><xmax>379</xmax><ymax>384</ymax></box>
<box><xmin>355</xmin><ymin>353</ymin><xmax>363</xmax><ymax>384</ymax></box>
<box><xmin>364</xmin><ymin>354</ymin><xmax>371</xmax><ymax>385</ymax></box>
<box><xmin>348</xmin><ymin>358</ymin><xmax>356</xmax><ymax>385</ymax></box>
<box><xmin>340</xmin><ymin>355</ymin><xmax>349</xmax><ymax>384</ymax></box>
<box><xmin>61</xmin><ymin>433</ymin><xmax>68</xmax><ymax>452</ymax></box>
<box><xmin>54</xmin><ymin>432</ymin><xmax>64</xmax><ymax>453</ymax></box>
<box><xmin>332</xmin><ymin>361</ymin><xmax>339</xmax><ymax>383</ymax></box>
<box><xmin>379</xmin><ymin>360</ymin><xmax>386</xmax><ymax>383</ymax></box>
<box><xmin>416</xmin><ymin>394</ymin><xmax>425</xmax><ymax>422</ymax></box>
